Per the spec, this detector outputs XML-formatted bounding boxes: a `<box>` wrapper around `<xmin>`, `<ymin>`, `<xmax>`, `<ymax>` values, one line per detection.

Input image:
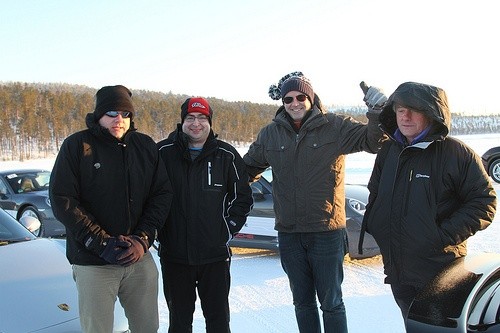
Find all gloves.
<box><xmin>360</xmin><ymin>81</ymin><xmax>387</xmax><ymax>108</ymax></box>
<box><xmin>115</xmin><ymin>235</ymin><xmax>144</xmax><ymax>267</ymax></box>
<box><xmin>100</xmin><ymin>236</ymin><xmax>131</xmax><ymax>263</ymax></box>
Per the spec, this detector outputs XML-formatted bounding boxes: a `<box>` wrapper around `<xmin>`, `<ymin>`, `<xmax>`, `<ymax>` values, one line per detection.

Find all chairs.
<box><xmin>20</xmin><ymin>176</ymin><xmax>36</xmax><ymax>192</ymax></box>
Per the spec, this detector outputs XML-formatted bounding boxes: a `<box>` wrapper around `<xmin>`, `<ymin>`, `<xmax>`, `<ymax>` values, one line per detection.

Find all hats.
<box><xmin>269</xmin><ymin>72</ymin><xmax>313</xmax><ymax>104</ymax></box>
<box><xmin>94</xmin><ymin>85</ymin><xmax>134</xmax><ymax>122</ymax></box>
<box><xmin>181</xmin><ymin>97</ymin><xmax>213</xmax><ymax>119</ymax></box>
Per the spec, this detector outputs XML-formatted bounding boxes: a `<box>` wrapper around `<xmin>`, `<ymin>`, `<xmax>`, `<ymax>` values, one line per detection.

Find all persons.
<box><xmin>242</xmin><ymin>73</ymin><xmax>389</xmax><ymax>333</ymax></box>
<box><xmin>156</xmin><ymin>97</ymin><xmax>253</xmax><ymax>333</ymax></box>
<box><xmin>357</xmin><ymin>82</ymin><xmax>498</xmax><ymax>331</ymax></box>
<box><xmin>47</xmin><ymin>84</ymin><xmax>173</xmax><ymax>333</ymax></box>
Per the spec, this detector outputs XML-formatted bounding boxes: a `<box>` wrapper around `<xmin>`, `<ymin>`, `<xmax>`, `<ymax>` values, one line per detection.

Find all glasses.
<box><xmin>284</xmin><ymin>94</ymin><xmax>308</xmax><ymax>104</ymax></box>
<box><xmin>186</xmin><ymin>115</ymin><xmax>207</xmax><ymax>121</ymax></box>
<box><xmin>105</xmin><ymin>110</ymin><xmax>131</xmax><ymax>118</ymax></box>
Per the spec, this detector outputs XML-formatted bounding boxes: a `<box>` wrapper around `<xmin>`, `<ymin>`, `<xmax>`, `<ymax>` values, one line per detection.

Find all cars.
<box><xmin>0</xmin><ymin>206</ymin><xmax>130</xmax><ymax>333</ymax></box>
<box><xmin>228</xmin><ymin>167</ymin><xmax>382</xmax><ymax>261</ymax></box>
<box><xmin>473</xmin><ymin>137</ymin><xmax>500</xmax><ymax>184</ymax></box>
<box><xmin>402</xmin><ymin>249</ymin><xmax>500</xmax><ymax>333</ymax></box>
<box><xmin>0</xmin><ymin>166</ymin><xmax>66</xmax><ymax>240</ymax></box>
